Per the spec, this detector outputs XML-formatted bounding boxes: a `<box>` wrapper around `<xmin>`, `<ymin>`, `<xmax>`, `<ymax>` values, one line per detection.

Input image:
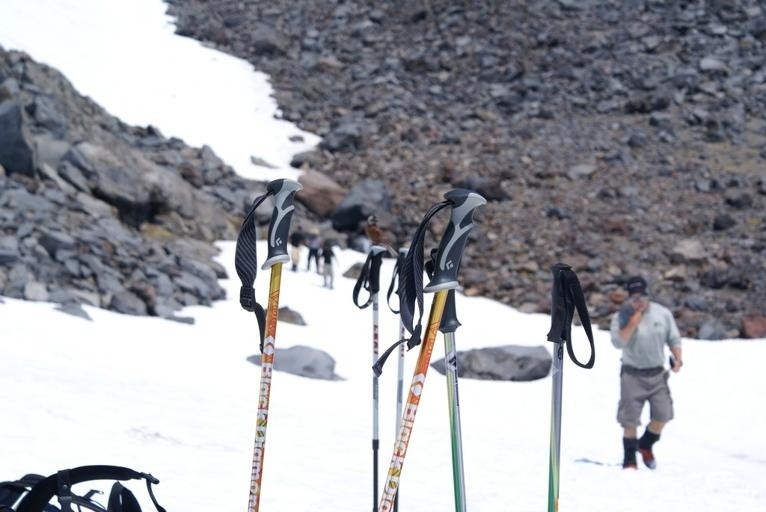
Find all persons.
<box><xmin>609</xmin><ymin>276</ymin><xmax>683</xmax><ymax>469</ymax></box>
<box><xmin>290</xmin><ymin>225</ymin><xmax>334</xmax><ymax>290</ymax></box>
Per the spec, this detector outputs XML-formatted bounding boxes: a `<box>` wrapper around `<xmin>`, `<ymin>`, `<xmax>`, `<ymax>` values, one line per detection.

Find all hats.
<box><xmin>625</xmin><ymin>276</ymin><xmax>653</xmax><ymax>296</ymax></box>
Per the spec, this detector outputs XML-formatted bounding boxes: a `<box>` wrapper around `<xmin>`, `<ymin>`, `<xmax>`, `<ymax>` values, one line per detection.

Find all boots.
<box><xmin>638</xmin><ymin>425</ymin><xmax>660</xmax><ymax>470</ymax></box>
<box><xmin>621</xmin><ymin>437</ymin><xmax>638</xmax><ymax>471</ymax></box>
